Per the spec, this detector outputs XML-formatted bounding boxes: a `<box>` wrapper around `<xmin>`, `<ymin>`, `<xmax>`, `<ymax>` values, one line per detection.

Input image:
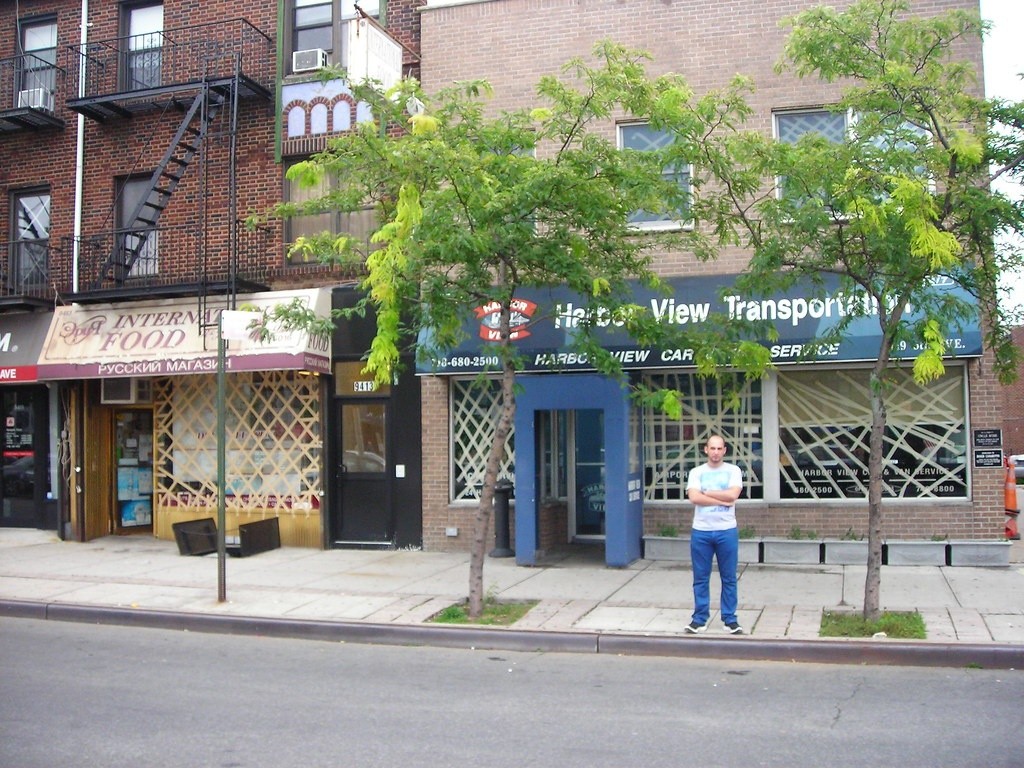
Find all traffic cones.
<box><xmin>1005</xmin><ymin>464</ymin><xmax>1021</xmax><ymax>513</ymax></box>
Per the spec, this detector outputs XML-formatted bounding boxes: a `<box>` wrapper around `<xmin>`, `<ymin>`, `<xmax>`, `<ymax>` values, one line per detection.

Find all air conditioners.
<box><xmin>18</xmin><ymin>88</ymin><xmax>52</xmax><ymax>112</ymax></box>
<box><xmin>292</xmin><ymin>49</ymin><xmax>329</xmax><ymax>73</ymax></box>
<box><xmin>100</xmin><ymin>377</ymin><xmax>152</xmax><ymax>404</ymax></box>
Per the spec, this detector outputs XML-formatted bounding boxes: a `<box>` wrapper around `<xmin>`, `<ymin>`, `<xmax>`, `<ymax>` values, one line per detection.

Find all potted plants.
<box><xmin>947</xmin><ymin>536</ymin><xmax>1013</xmax><ymax>567</ymax></box>
<box><xmin>642</xmin><ymin>524</ymin><xmax>691</xmax><ymax>562</ymax></box>
<box><xmin>884</xmin><ymin>534</ymin><xmax>951</xmax><ymax>567</ymax></box>
<box><xmin>761</xmin><ymin>527</ymin><xmax>823</xmax><ymax>565</ymax></box>
<box><xmin>821</xmin><ymin>526</ymin><xmax>869</xmax><ymax>565</ymax></box>
<box><xmin>711</xmin><ymin>526</ymin><xmax>761</xmax><ymax>563</ymax></box>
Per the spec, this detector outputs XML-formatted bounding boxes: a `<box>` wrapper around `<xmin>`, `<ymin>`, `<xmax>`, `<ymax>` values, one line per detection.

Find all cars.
<box><xmin>658</xmin><ymin>444</ymin><xmax>966</xmax><ymax>478</ymax></box>
<box><xmin>342</xmin><ymin>451</ymin><xmax>385</xmax><ymax>472</ymax></box>
<box><xmin>2</xmin><ymin>451</ymin><xmax>51</xmax><ymax>497</ymax></box>
<box><xmin>1008</xmin><ymin>455</ymin><xmax>1024</xmax><ymax>479</ymax></box>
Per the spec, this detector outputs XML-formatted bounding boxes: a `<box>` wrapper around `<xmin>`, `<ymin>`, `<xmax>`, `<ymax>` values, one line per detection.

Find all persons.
<box><xmin>684</xmin><ymin>434</ymin><xmax>743</xmax><ymax>634</ymax></box>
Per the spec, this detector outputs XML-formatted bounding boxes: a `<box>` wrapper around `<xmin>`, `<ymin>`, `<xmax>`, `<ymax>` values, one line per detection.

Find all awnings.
<box><xmin>416</xmin><ymin>259</ymin><xmax>983</xmax><ymax>376</ymax></box>
<box><xmin>0</xmin><ymin>287</ymin><xmax>333</xmax><ymax>385</ymax></box>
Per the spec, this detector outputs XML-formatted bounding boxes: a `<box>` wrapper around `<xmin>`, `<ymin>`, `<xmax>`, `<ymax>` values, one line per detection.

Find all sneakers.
<box><xmin>686</xmin><ymin>622</ymin><xmax>706</xmax><ymax>633</ymax></box>
<box><xmin>724</xmin><ymin>624</ymin><xmax>744</xmax><ymax>635</ymax></box>
